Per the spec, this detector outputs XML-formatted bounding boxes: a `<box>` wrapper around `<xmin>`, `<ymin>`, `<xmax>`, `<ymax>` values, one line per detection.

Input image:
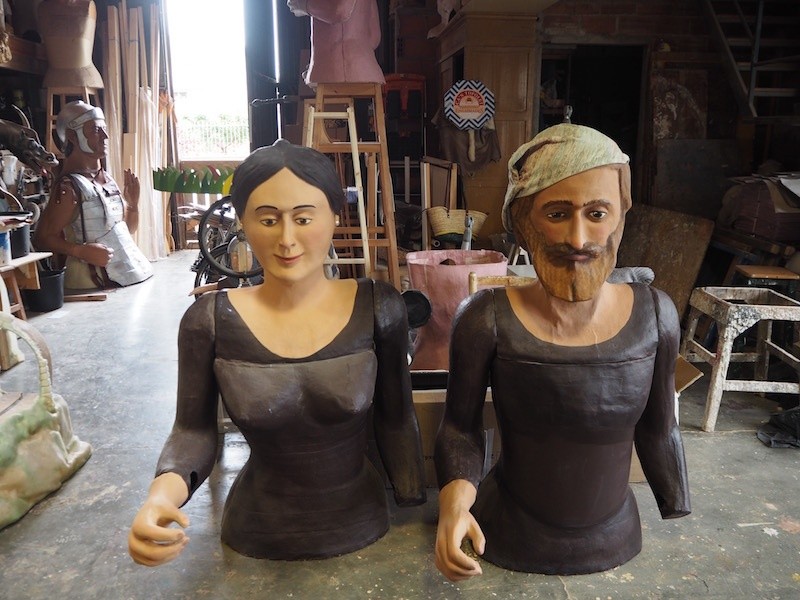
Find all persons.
<box><xmin>37</xmin><ymin>0</ymin><xmax>105</xmax><ymax>88</ymax></box>
<box><xmin>128</xmin><ymin>137</ymin><xmax>428</xmax><ymax>566</ymax></box>
<box><xmin>432</xmin><ymin>123</ymin><xmax>693</xmax><ymax>581</ymax></box>
<box><xmin>287</xmin><ymin>0</ymin><xmax>387</xmax><ymax>84</ymax></box>
<box><xmin>31</xmin><ymin>100</ymin><xmax>153</xmax><ymax>288</ymax></box>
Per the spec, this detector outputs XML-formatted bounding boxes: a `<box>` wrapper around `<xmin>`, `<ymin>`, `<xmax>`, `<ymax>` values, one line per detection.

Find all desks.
<box><xmin>0</xmin><ymin>252</ymin><xmax>53</xmax><ymax>320</ymax></box>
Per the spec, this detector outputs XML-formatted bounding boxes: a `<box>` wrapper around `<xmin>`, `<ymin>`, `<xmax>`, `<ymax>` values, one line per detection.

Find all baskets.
<box><xmin>426</xmin><ymin>162</ymin><xmax>488</xmax><ymax>241</ymax></box>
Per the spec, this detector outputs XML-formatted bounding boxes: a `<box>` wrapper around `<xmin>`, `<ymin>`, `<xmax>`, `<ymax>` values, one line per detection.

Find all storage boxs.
<box><xmin>386</xmin><ymin>354</ymin><xmax>704</xmax><ymax>488</ymax></box>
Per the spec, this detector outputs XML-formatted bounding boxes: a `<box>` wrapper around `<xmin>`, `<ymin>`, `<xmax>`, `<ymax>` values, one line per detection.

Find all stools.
<box><xmin>734</xmin><ymin>265</ymin><xmax>800</xmax><ymax>356</ymax></box>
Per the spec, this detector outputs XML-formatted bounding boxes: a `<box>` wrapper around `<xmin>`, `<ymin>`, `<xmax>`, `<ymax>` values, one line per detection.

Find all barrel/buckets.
<box><xmin>19</xmin><ymin>269</ymin><xmax>65</xmax><ymax>314</ymax></box>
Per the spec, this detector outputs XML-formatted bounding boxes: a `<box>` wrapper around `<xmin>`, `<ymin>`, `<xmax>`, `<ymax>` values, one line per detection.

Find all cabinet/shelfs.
<box><xmin>437</xmin><ymin>11</ymin><xmax>537</xmax><ymax>244</ymax></box>
